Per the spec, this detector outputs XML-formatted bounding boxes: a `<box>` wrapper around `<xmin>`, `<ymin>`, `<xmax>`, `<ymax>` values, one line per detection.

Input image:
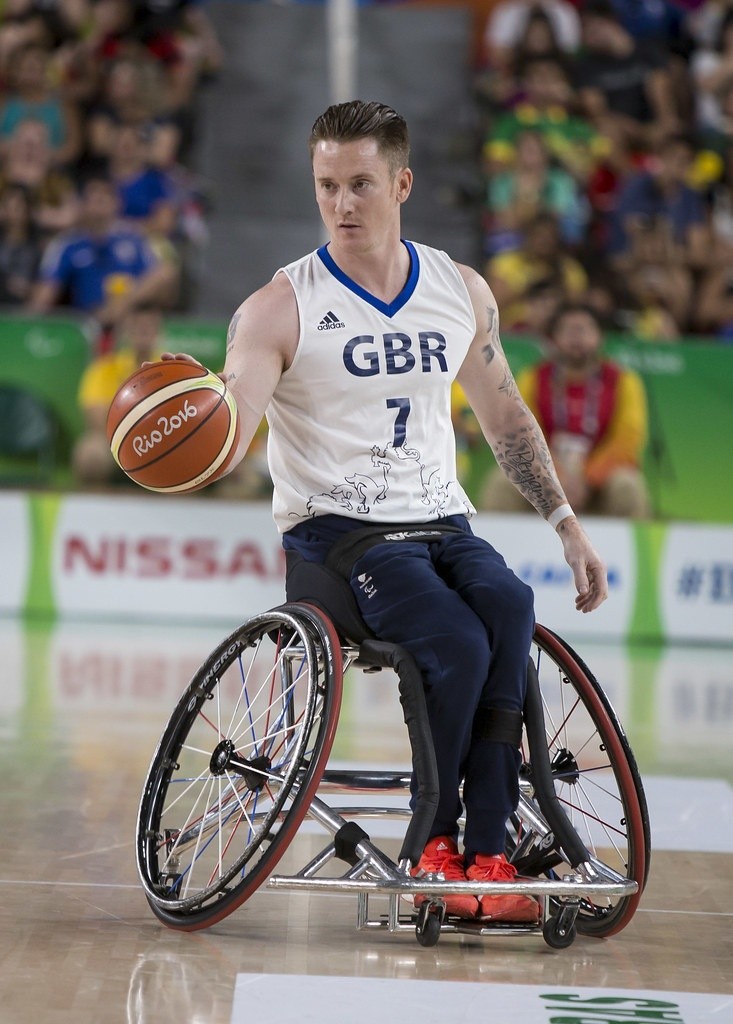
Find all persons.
<box><xmin>140</xmin><ymin>100</ymin><xmax>609</xmax><ymax>921</ymax></box>
<box><xmin>483</xmin><ymin>299</ymin><xmax>650</xmax><ymax>516</ymax></box>
<box><xmin>0</xmin><ymin>1</ymin><xmax>226</xmax><ymax>496</ymax></box>
<box><xmin>463</xmin><ymin>0</ymin><xmax>733</xmax><ymax>338</ymax></box>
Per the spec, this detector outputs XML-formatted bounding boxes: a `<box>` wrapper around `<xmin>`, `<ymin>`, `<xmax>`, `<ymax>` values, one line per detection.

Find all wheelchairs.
<box><xmin>134</xmin><ymin>550</ymin><xmax>652</xmax><ymax>948</ymax></box>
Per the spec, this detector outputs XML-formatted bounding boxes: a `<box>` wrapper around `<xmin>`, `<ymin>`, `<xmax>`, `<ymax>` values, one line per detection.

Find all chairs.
<box><xmin>0</xmin><ymin>389</ymin><xmax>60</xmax><ymax>487</ymax></box>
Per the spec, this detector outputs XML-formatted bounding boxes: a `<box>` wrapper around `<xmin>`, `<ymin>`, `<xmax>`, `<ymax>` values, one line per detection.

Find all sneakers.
<box><xmin>408</xmin><ymin>835</ymin><xmax>477</xmax><ymax>919</ymax></box>
<box><xmin>460</xmin><ymin>850</ymin><xmax>540</xmax><ymax>924</ymax></box>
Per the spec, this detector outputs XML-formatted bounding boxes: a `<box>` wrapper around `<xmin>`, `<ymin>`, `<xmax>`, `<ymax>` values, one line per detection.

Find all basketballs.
<box><xmin>103</xmin><ymin>359</ymin><xmax>241</xmax><ymax>495</ymax></box>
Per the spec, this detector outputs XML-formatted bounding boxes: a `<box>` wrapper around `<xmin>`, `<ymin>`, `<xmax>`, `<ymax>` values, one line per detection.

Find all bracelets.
<box><xmin>546</xmin><ymin>502</ymin><xmax>577</xmax><ymax>534</ymax></box>
